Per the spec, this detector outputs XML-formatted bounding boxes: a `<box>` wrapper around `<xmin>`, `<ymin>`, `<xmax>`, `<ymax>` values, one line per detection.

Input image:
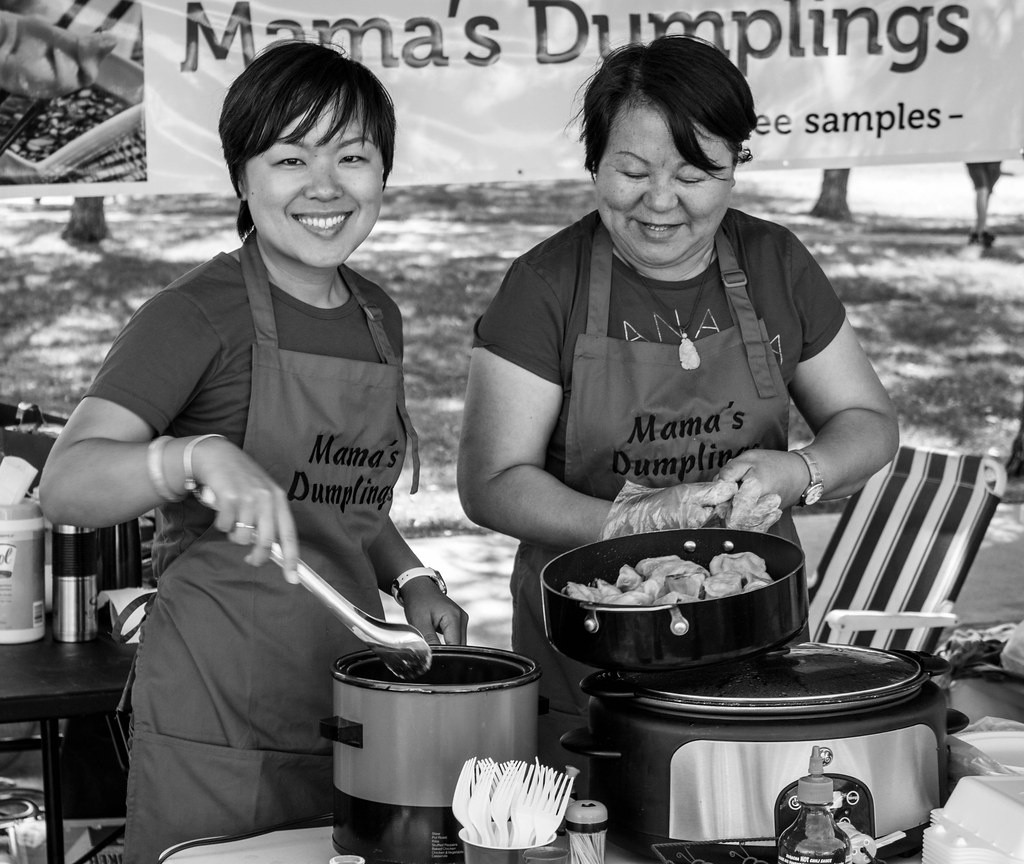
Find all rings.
<box><xmin>235</xmin><ymin>522</ymin><xmax>254</xmax><ymax>530</ymax></box>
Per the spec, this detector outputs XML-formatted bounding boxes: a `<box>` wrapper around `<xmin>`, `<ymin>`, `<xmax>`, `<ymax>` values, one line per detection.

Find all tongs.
<box><xmin>249</xmin><ymin>539</ymin><xmax>431</xmax><ymax>678</ymax></box>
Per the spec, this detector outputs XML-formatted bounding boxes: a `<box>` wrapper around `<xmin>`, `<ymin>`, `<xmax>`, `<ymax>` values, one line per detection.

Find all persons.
<box><xmin>456</xmin><ymin>32</ymin><xmax>900</xmax><ymax>803</ymax></box>
<box><xmin>968</xmin><ymin>162</ymin><xmax>1001</xmax><ymax>248</ymax></box>
<box><xmin>38</xmin><ymin>42</ymin><xmax>470</xmax><ymax>864</ymax></box>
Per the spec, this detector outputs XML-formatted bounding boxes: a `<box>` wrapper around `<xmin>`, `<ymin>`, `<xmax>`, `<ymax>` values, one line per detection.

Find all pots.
<box><xmin>578</xmin><ymin>643</ymin><xmax>967</xmax><ymax>844</ymax></box>
<box><xmin>327</xmin><ymin>645</ymin><xmax>539</xmax><ymax>864</ymax></box>
<box><xmin>536</xmin><ymin>479</ymin><xmax>808</xmax><ymax>668</ymax></box>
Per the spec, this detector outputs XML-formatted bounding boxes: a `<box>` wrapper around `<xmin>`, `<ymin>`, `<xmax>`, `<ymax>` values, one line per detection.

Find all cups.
<box><xmin>523</xmin><ymin>847</ymin><xmax>570</xmax><ymax>864</ymax></box>
<box><xmin>458</xmin><ymin>826</ymin><xmax>557</xmax><ymax>864</ymax></box>
<box><xmin>0</xmin><ymin>502</ymin><xmax>43</xmax><ymax>645</ymax></box>
<box><xmin>54</xmin><ymin>523</ymin><xmax>101</xmax><ymax>642</ymax></box>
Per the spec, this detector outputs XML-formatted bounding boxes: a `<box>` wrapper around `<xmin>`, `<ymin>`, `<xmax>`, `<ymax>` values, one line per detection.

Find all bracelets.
<box><xmin>182</xmin><ymin>434</ymin><xmax>226</xmax><ymax>492</ymax></box>
<box><xmin>146</xmin><ymin>436</ymin><xmax>188</xmax><ymax>502</ymax></box>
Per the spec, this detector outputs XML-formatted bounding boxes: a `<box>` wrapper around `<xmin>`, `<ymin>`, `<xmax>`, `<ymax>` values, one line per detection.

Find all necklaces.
<box><xmin>623</xmin><ymin>240</ymin><xmax>715</xmax><ymax>370</ymax></box>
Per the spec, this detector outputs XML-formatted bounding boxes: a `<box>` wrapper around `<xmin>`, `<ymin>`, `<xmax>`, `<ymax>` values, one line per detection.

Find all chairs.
<box><xmin>806</xmin><ymin>446</ymin><xmax>1009</xmax><ymax>656</ymax></box>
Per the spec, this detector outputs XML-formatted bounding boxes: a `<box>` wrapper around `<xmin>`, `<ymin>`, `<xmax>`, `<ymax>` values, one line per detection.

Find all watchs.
<box><xmin>391</xmin><ymin>567</ymin><xmax>447</xmax><ymax>606</ymax></box>
<box><xmin>790</xmin><ymin>449</ymin><xmax>824</xmax><ymax>507</ymax></box>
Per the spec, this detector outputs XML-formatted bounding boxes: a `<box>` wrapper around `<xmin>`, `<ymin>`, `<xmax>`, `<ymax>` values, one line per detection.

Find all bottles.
<box><xmin>773</xmin><ymin>747</ymin><xmax>850</xmax><ymax>864</ymax></box>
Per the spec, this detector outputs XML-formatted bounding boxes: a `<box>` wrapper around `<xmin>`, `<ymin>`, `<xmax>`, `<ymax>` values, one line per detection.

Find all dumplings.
<box><xmin>568</xmin><ymin>553</ymin><xmax>772</xmax><ymax>608</ymax></box>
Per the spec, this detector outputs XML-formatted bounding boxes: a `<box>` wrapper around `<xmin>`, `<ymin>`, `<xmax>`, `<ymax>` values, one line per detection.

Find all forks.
<box><xmin>449</xmin><ymin>758</ymin><xmax>575</xmax><ymax>844</ymax></box>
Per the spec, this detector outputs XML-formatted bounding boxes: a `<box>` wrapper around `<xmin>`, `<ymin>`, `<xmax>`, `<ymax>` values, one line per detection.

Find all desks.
<box><xmin>0</xmin><ymin>610</ymin><xmax>139</xmax><ymax>864</ymax></box>
<box><xmin>159</xmin><ymin>826</ymin><xmax>921</xmax><ymax>864</ymax></box>
